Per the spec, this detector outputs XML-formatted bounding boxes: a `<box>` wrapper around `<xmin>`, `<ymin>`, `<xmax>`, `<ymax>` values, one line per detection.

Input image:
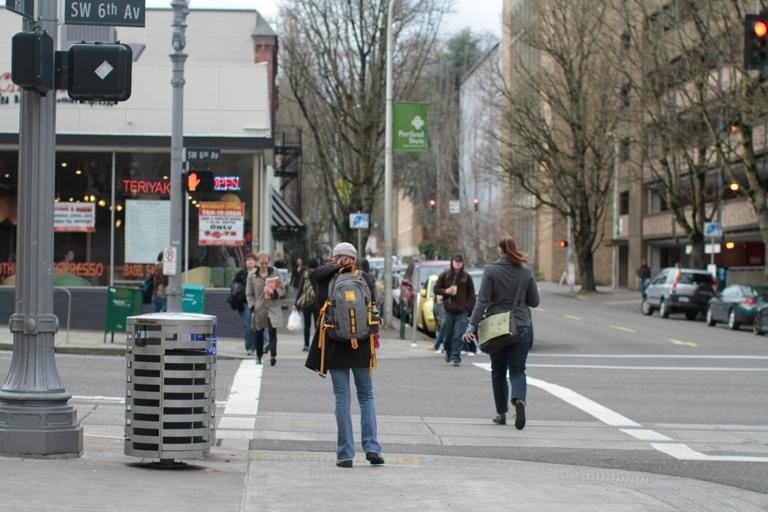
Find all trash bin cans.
<box><xmin>126</xmin><ymin>313</ymin><xmax>217</xmax><ymax>468</ymax></box>
<box><xmin>182</xmin><ymin>285</ymin><xmax>206</xmax><ymax>314</ymax></box>
<box><xmin>104</xmin><ymin>286</ymin><xmax>144</xmax><ymax>343</ymax></box>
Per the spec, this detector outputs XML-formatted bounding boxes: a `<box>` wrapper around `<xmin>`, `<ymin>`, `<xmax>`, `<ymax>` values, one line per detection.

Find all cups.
<box><xmin>451</xmin><ymin>285</ymin><xmax>457</xmax><ymax>295</ymax></box>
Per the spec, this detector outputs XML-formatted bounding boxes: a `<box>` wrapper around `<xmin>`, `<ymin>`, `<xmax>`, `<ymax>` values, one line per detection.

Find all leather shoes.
<box><xmin>492</xmin><ymin>414</ymin><xmax>506</xmax><ymax>425</ymax></box>
<box><xmin>514</xmin><ymin>402</ymin><xmax>525</xmax><ymax>430</ymax></box>
<box><xmin>337</xmin><ymin>460</ymin><xmax>354</xmax><ymax>467</ymax></box>
<box><xmin>303</xmin><ymin>345</ymin><xmax>310</xmax><ymax>352</ymax></box>
<box><xmin>365</xmin><ymin>451</ymin><xmax>384</xmax><ymax>465</ymax></box>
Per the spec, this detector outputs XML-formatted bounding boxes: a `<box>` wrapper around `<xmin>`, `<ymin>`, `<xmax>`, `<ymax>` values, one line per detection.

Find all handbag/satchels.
<box><xmin>296</xmin><ymin>270</ymin><xmax>316</xmax><ymax>309</ymax></box>
<box><xmin>477</xmin><ymin>310</ymin><xmax>519</xmax><ymax>355</ymax></box>
<box><xmin>227</xmin><ymin>285</ymin><xmax>248</xmax><ymax>311</ymax></box>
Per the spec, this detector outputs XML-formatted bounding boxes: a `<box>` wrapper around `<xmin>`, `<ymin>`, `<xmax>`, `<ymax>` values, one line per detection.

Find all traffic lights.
<box><xmin>473</xmin><ymin>197</ymin><xmax>479</xmax><ymax>212</ymax></box>
<box><xmin>560</xmin><ymin>239</ymin><xmax>568</xmax><ymax>247</ymax></box>
<box><xmin>743</xmin><ymin>13</ymin><xmax>768</xmax><ymax>70</ymax></box>
<box><xmin>184</xmin><ymin>170</ymin><xmax>214</xmax><ymax>194</ymax></box>
<box><xmin>429</xmin><ymin>200</ymin><xmax>436</xmax><ymax>214</ymax></box>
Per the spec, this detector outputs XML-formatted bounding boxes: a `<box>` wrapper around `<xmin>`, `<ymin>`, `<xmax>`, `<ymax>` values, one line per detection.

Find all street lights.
<box><xmin>605</xmin><ymin>131</ymin><xmax>618</xmax><ymax>290</ymax></box>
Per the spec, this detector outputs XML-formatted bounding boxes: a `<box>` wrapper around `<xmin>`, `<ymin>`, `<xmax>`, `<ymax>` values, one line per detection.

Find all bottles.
<box><xmin>372</xmin><ymin>302</ymin><xmax>380</xmax><ymax>317</ymax></box>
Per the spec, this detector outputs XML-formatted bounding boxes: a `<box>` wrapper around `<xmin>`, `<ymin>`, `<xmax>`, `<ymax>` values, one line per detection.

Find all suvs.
<box><xmin>640</xmin><ymin>267</ymin><xmax>717</xmax><ymax>320</ymax></box>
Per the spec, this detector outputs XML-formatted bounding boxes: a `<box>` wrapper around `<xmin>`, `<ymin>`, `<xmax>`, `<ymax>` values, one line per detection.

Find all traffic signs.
<box><xmin>63</xmin><ymin>1</ymin><xmax>146</xmax><ymax>29</ymax></box>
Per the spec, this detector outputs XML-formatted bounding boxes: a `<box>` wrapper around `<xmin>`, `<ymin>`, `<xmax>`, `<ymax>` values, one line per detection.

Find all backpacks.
<box><xmin>139</xmin><ymin>273</ymin><xmax>156</xmax><ymax>305</ymax></box>
<box><xmin>316</xmin><ymin>264</ymin><xmax>383</xmax><ymax>378</ymax></box>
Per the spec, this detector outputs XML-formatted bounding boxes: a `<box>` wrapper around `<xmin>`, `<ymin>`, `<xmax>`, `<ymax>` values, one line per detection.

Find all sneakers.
<box><xmin>271</xmin><ymin>359</ymin><xmax>276</xmax><ymax>366</ymax></box>
<box><xmin>256</xmin><ymin>356</ymin><xmax>262</xmax><ymax>364</ymax></box>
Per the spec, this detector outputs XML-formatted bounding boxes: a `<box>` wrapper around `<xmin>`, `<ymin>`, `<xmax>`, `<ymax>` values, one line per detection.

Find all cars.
<box><xmin>704</xmin><ymin>283</ymin><xmax>768</xmax><ymax>333</ymax></box>
<box><xmin>364</xmin><ymin>253</ymin><xmax>487</xmax><ymax>337</ymax></box>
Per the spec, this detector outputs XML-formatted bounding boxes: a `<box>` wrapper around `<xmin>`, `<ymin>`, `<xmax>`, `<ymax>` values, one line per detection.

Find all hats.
<box><xmin>308</xmin><ymin>259</ymin><xmax>318</xmax><ymax>269</ymax></box>
<box><xmin>454</xmin><ymin>255</ymin><xmax>462</xmax><ymax>261</ymax></box>
<box><xmin>332</xmin><ymin>242</ymin><xmax>358</xmax><ymax>258</ymax></box>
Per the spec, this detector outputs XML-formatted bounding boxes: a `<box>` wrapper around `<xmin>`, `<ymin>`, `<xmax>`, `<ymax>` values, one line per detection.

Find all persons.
<box><xmin>61</xmin><ymin>249</ymin><xmax>75</xmax><ymax>263</ymax></box>
<box><xmin>150</xmin><ymin>250</ymin><xmax>169</xmax><ymax>313</ymax></box>
<box><xmin>433</xmin><ymin>254</ymin><xmax>477</xmax><ymax>366</ymax></box>
<box><xmin>460</xmin><ymin>235</ymin><xmax>540</xmax><ymax>430</ymax></box>
<box><xmin>637</xmin><ymin>257</ymin><xmax>650</xmax><ymax>302</ymax></box>
<box><xmin>226</xmin><ymin>245</ymin><xmax>378</xmax><ymax>367</ymax></box>
<box><xmin>306</xmin><ymin>240</ymin><xmax>385</xmax><ymax>468</ymax></box>
